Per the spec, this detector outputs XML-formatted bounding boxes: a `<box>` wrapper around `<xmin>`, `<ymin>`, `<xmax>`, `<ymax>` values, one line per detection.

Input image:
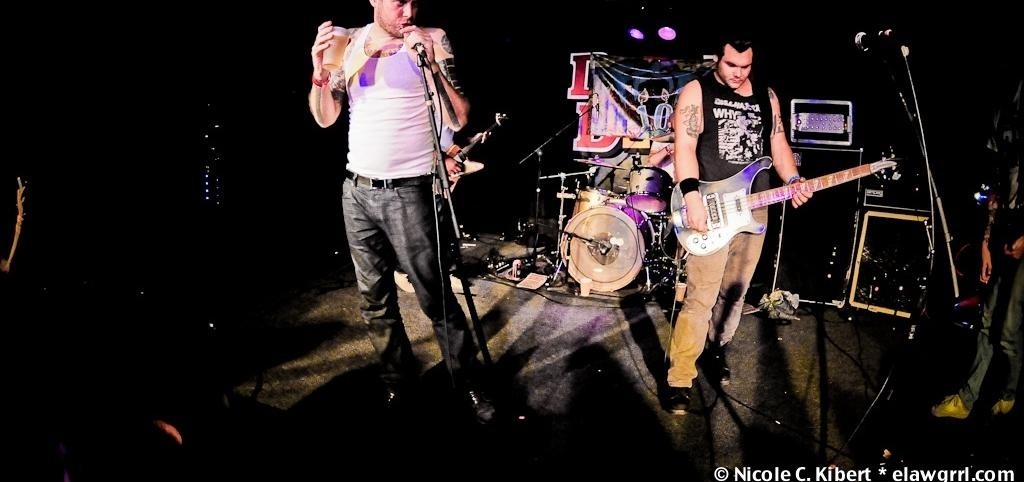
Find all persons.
<box><xmin>433</xmin><ymin>126</ymin><xmax>464</xmax><ymax>175</ymax></box>
<box><xmin>307</xmin><ymin>0</ymin><xmax>507</xmax><ymax>429</ymax></box>
<box><xmin>660</xmin><ymin>29</ymin><xmax>814</xmax><ymax>416</ymax></box>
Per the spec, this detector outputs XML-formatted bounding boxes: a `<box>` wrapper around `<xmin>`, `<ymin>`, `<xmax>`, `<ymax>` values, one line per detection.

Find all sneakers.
<box><xmin>930</xmin><ymin>394</ymin><xmax>969</xmax><ymax>419</ymax></box>
<box><xmin>990</xmin><ymin>399</ymin><xmax>1015</xmax><ymax>415</ymax></box>
<box><xmin>449</xmin><ymin>275</ymin><xmax>466</xmax><ymax>296</ymax></box>
<box><xmin>392</xmin><ymin>270</ymin><xmax>415</xmax><ymax>292</ymax></box>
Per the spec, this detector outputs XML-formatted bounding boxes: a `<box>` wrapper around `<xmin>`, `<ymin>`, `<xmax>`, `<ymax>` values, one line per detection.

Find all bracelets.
<box><xmin>679</xmin><ymin>178</ymin><xmax>700</xmax><ymax>192</ymax></box>
<box><xmin>310</xmin><ymin>71</ymin><xmax>332</xmax><ymax>87</ymax></box>
<box><xmin>787</xmin><ymin>175</ymin><xmax>801</xmax><ymax>185</ymax></box>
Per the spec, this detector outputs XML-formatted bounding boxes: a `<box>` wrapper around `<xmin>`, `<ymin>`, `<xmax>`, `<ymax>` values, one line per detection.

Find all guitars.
<box><xmin>670</xmin><ymin>146</ymin><xmax>902</xmax><ymax>256</ymax></box>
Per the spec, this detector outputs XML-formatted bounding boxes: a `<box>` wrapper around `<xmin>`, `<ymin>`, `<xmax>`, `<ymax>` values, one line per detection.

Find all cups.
<box><xmin>674</xmin><ymin>283</ymin><xmax>687</xmax><ymax>302</ymax></box>
<box><xmin>321</xmin><ymin>26</ymin><xmax>351</xmax><ymax>72</ymax></box>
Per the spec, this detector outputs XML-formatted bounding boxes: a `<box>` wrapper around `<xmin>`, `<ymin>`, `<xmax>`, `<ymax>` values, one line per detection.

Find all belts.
<box><xmin>346</xmin><ymin>171</ymin><xmax>427</xmax><ymax>189</ymax></box>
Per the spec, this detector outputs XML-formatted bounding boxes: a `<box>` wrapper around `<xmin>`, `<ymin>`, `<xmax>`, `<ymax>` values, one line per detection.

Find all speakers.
<box><xmin>771</xmin><ymin>146</ymin><xmax>863</xmax><ymax>311</ymax></box>
<box><xmin>847</xmin><ymin>210</ymin><xmax>929</xmax><ymax>321</ymax></box>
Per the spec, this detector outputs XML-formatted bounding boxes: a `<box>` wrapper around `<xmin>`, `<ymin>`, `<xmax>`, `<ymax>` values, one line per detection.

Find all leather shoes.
<box><xmin>379</xmin><ymin>388</ymin><xmax>411</xmax><ymax>411</ymax></box>
<box><xmin>706</xmin><ymin>350</ymin><xmax>731</xmax><ymax>385</ymax></box>
<box><xmin>668</xmin><ymin>387</ymin><xmax>693</xmax><ymax>416</ymax></box>
<box><xmin>456</xmin><ymin>385</ymin><xmax>496</xmax><ymax>424</ymax></box>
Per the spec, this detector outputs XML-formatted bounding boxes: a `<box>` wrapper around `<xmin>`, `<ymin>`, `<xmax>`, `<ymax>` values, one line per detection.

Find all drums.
<box><xmin>559</xmin><ymin>199</ymin><xmax>654</xmax><ymax>295</ymax></box>
<box><xmin>572</xmin><ymin>185</ymin><xmax>629</xmax><ymax>216</ymax></box>
<box><xmin>626</xmin><ymin>167</ymin><xmax>674</xmax><ymax>216</ymax></box>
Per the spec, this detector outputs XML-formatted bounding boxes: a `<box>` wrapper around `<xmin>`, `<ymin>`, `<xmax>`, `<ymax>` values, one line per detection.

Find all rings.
<box><xmin>314</xmin><ymin>41</ymin><xmax>318</xmax><ymax>48</ymax></box>
<box><xmin>448</xmin><ymin>173</ymin><xmax>451</xmax><ymax>174</ymax></box>
<box><xmin>694</xmin><ymin>224</ymin><xmax>698</xmax><ymax>227</ymax></box>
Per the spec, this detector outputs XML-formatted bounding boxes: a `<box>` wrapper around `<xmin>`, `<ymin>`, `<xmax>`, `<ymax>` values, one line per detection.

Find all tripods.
<box><xmin>496</xmin><ymin>105</ymin><xmax>594</xmax><ymax>290</ymax></box>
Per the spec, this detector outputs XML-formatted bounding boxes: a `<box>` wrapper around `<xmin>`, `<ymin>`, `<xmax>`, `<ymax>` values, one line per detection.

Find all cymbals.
<box><xmin>575</xmin><ymin>157</ymin><xmax>625</xmax><ymax>170</ymax></box>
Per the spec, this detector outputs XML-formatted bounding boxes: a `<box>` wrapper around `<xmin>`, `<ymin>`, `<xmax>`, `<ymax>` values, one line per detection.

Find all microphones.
<box><xmin>402</xmin><ymin>24</ymin><xmax>427</xmax><ymax>59</ymax></box>
<box><xmin>598</xmin><ymin>239</ymin><xmax>612</xmax><ymax>256</ymax></box>
<box><xmin>596</xmin><ymin>93</ymin><xmax>599</xmax><ymax>118</ymax></box>
<box><xmin>855</xmin><ymin>32</ymin><xmax>909</xmax><ymax>62</ymax></box>
